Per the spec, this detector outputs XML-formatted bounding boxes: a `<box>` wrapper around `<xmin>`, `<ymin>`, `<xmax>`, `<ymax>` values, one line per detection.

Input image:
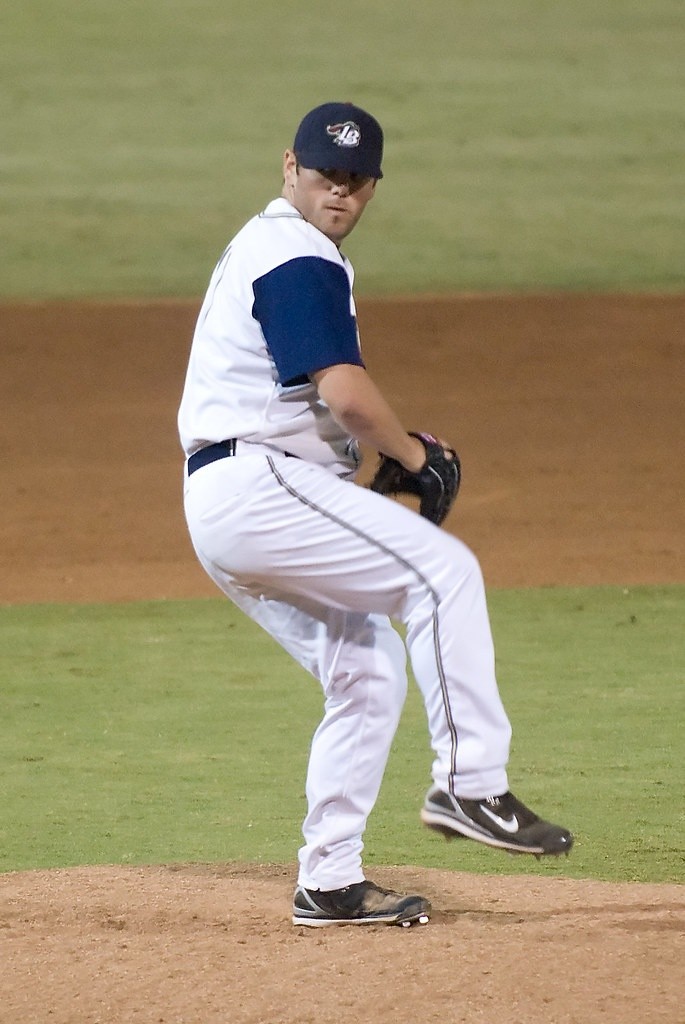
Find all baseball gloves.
<box><xmin>370</xmin><ymin>433</ymin><xmax>461</xmax><ymax>526</ymax></box>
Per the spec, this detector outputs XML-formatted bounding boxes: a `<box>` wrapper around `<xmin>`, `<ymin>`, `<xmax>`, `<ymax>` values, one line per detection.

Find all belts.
<box><xmin>188</xmin><ymin>437</ymin><xmax>303</xmax><ymax>476</ymax></box>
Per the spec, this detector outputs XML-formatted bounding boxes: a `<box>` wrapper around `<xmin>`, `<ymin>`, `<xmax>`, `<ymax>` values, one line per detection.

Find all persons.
<box><xmin>177</xmin><ymin>102</ymin><xmax>572</xmax><ymax>927</ymax></box>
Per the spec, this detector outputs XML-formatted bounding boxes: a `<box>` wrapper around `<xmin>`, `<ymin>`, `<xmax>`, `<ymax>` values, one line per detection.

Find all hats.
<box><xmin>293</xmin><ymin>102</ymin><xmax>383</xmax><ymax>180</ymax></box>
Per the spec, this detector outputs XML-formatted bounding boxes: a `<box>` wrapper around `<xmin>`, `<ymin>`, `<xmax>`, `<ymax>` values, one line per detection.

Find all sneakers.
<box><xmin>292</xmin><ymin>879</ymin><xmax>432</xmax><ymax>928</ymax></box>
<box><xmin>419</xmin><ymin>785</ymin><xmax>574</xmax><ymax>859</ymax></box>
